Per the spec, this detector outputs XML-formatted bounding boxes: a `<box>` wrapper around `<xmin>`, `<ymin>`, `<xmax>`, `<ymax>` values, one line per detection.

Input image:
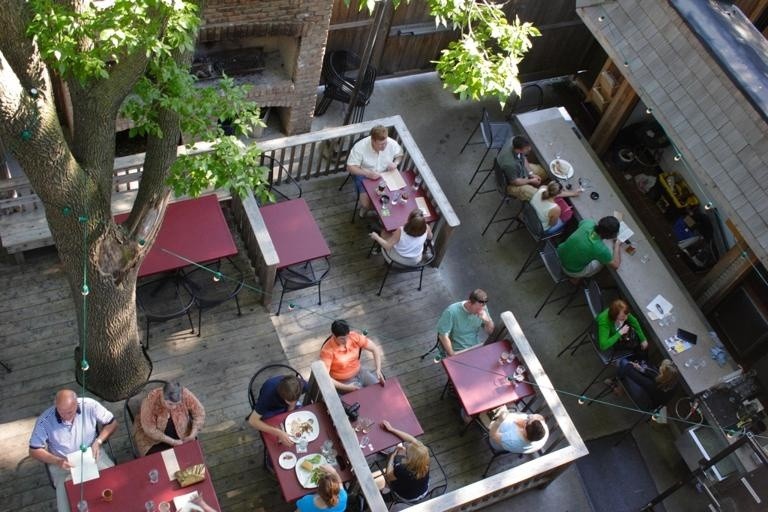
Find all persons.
<box><xmin>295</xmin><ymin>464</ymin><xmax>347</xmax><ymax>512</ymax></box>
<box><xmin>531</xmin><ymin>179</ymin><xmax>582</xmax><ymax>235</ymax></box>
<box><xmin>320</xmin><ymin>320</ymin><xmax>385</xmax><ymax>392</ymax></box>
<box><xmin>132</xmin><ymin>381</ymin><xmax>207</xmax><ymax>456</ymax></box>
<box><xmin>461</xmin><ymin>403</ymin><xmax>549</xmax><ymax>452</ymax></box>
<box><xmin>29</xmin><ymin>390</ymin><xmax>118</xmax><ymax>511</ymax></box>
<box><xmin>380</xmin><ymin>420</ymin><xmax>430</xmax><ymax>503</ymax></box>
<box><xmin>558</xmin><ymin>211</ymin><xmax>620</xmax><ymax>284</ymax></box>
<box><xmin>248</xmin><ymin>374</ymin><xmax>308</xmax><ymax>447</ymax></box>
<box><xmin>495</xmin><ymin>134</ymin><xmax>548</xmax><ymax>201</ymax></box>
<box><xmin>604</xmin><ymin>359</ymin><xmax>678</xmax><ymax>414</ymax></box>
<box><xmin>346</xmin><ymin>125</ymin><xmax>404</xmax><ymax>217</ymax></box>
<box><xmin>596</xmin><ymin>299</ymin><xmax>648</xmax><ymax>358</ymax></box>
<box><xmin>437</xmin><ymin>288</ymin><xmax>494</xmax><ymax>358</ymax></box>
<box><xmin>369</xmin><ymin>208</ymin><xmax>434</xmax><ymax>266</ymax></box>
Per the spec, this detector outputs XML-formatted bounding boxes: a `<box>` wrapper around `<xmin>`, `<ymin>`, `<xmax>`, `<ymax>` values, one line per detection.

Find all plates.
<box><xmin>550</xmin><ymin>158</ymin><xmax>574</xmax><ymax>180</ymax></box>
<box><xmin>285</xmin><ymin>410</ymin><xmax>320</xmax><ymax>443</ymax></box>
<box><xmin>278</xmin><ymin>451</ymin><xmax>297</xmax><ymax>469</ymax></box>
<box><xmin>295</xmin><ymin>452</ymin><xmax>327</xmax><ymax>489</ymax></box>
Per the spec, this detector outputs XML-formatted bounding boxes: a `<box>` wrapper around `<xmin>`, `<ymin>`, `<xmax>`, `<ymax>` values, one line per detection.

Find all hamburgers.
<box><xmin>302</xmin><ymin>461</ymin><xmax>313</xmax><ymax>470</ymax></box>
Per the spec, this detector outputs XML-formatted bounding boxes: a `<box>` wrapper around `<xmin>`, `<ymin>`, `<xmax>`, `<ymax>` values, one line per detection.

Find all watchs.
<box><xmin>528</xmin><ymin>171</ymin><xmax>533</xmax><ymax>176</ymax></box>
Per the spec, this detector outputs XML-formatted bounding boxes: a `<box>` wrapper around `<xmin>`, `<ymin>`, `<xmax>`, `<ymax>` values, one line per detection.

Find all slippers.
<box><xmin>604</xmin><ymin>378</ymin><xmax>623</xmax><ymax>397</ymax></box>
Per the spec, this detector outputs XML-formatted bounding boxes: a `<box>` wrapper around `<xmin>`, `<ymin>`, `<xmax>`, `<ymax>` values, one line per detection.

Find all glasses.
<box><xmin>410</xmin><ymin>213</ymin><xmax>423</xmax><ymax>218</ymax></box>
<box><xmin>478</xmin><ymin>299</ymin><xmax>489</xmax><ymax>303</ymax></box>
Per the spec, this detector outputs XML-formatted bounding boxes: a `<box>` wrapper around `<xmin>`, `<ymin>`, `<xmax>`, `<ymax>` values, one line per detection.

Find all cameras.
<box><xmin>341</xmin><ymin>400</ymin><xmax>360</xmax><ymax>420</ymax></box>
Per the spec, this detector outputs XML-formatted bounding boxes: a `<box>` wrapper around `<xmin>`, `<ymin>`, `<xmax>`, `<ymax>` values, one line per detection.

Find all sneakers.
<box><xmin>370</xmin><ymin>246</ymin><xmax>382</xmax><ymax>255</ymax></box>
<box><xmin>358</xmin><ymin>207</ymin><xmax>368</xmax><ymax>219</ymax></box>
<box><xmin>366</xmin><ymin>209</ymin><xmax>378</xmax><ymax>217</ymax></box>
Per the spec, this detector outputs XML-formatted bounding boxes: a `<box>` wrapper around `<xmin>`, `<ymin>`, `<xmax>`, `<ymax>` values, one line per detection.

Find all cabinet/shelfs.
<box><xmin>584</xmin><ymin>56</ymin><xmax>625</xmax><ymax>116</ymax></box>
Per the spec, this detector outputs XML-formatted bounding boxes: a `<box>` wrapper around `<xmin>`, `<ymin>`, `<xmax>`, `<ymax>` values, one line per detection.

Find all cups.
<box><xmin>363</xmin><ymin>417</ymin><xmax>375</xmax><ymax>433</ymax></box>
<box><xmin>149</xmin><ymin>470</ymin><xmax>158</xmax><ymax>483</ymax></box>
<box><xmin>412</xmin><ymin>177</ymin><xmax>421</xmax><ymax>190</ymax></box>
<box><xmin>392</xmin><ymin>190</ymin><xmax>399</xmax><ymax>205</ymax></box>
<box><xmin>563</xmin><ymin>167</ymin><xmax>569</xmax><ymax>182</ymax></box>
<box><xmin>144</xmin><ymin>500</ymin><xmax>155</xmax><ymax>512</ymax></box>
<box><xmin>159</xmin><ymin>501</ymin><xmax>170</xmax><ymax>512</ymax></box>
<box><xmin>327</xmin><ymin>449</ymin><xmax>337</xmax><ymax>462</ymax></box>
<box><xmin>359</xmin><ymin>436</ymin><xmax>369</xmax><ymax>448</ymax></box>
<box><xmin>694</xmin><ymin>358</ymin><xmax>707</xmax><ymax>369</ymax></box>
<box><xmin>401</xmin><ymin>192</ymin><xmax>409</xmax><ymax>205</ymax></box>
<box><xmin>513</xmin><ymin>365</ymin><xmax>525</xmax><ymax>377</ymax></box>
<box><xmin>554</xmin><ymin>151</ymin><xmax>561</xmax><ymax>159</ymax></box>
<box><xmin>515</xmin><ymin>376</ymin><xmax>524</xmax><ymax>385</ymax></box>
<box><xmin>377</xmin><ymin>181</ymin><xmax>386</xmax><ymax>195</ymax></box>
<box><xmin>684</xmin><ymin>357</ymin><xmax>695</xmax><ymax>366</ymax></box>
<box><xmin>354</xmin><ymin>416</ymin><xmax>364</xmax><ymax>432</ymax></box>
<box><xmin>507</xmin><ymin>350</ymin><xmax>516</xmax><ymax>363</ymax></box>
<box><xmin>626</xmin><ymin>243</ymin><xmax>637</xmax><ymax>255</ymax></box>
<box><xmin>322</xmin><ymin>440</ymin><xmax>333</xmax><ymax>454</ymax></box>
<box><xmin>498</xmin><ymin>351</ymin><xmax>509</xmax><ymax>365</ymax></box>
<box><xmin>379</xmin><ymin>196</ymin><xmax>390</xmax><ymax>209</ymax></box>
<box><xmin>657</xmin><ymin>313</ymin><xmax>677</xmax><ymax>326</ymax></box>
<box><xmin>100</xmin><ymin>490</ymin><xmax>113</xmax><ymax>502</ymax></box>
<box><xmin>299</xmin><ymin>439</ymin><xmax>308</xmax><ymax>451</ymax></box>
<box><xmin>640</xmin><ymin>254</ymin><xmax>649</xmax><ymax>263</ymax></box>
<box><xmin>78</xmin><ymin>501</ymin><xmax>89</xmax><ymax>512</ymax></box>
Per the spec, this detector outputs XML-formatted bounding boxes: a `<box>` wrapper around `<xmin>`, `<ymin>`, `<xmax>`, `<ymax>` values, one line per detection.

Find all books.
<box><xmin>63</xmin><ymin>448</ymin><xmax>101</xmax><ymax>483</ymax></box>
<box><xmin>617</xmin><ymin>220</ymin><xmax>634</xmax><ymax>242</ymax></box>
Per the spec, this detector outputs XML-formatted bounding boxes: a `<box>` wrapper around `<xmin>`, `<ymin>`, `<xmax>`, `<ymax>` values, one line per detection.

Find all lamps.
<box><xmin>361</xmin><ymin>330</ymin><xmax>368</xmax><ymax>338</ymax></box>
<box><xmin>137</xmin><ymin>240</ymin><xmax>144</xmax><ymax>248</ymax></box>
<box><xmin>645</xmin><ymin>107</ymin><xmax>652</xmax><ymax>114</ymax></box>
<box><xmin>727</xmin><ymin>430</ymin><xmax>735</xmax><ymax>439</ymax></box>
<box><xmin>81</xmin><ymin>445</ymin><xmax>91</xmax><ymax>459</ymax></box>
<box><xmin>212</xmin><ymin>272</ymin><xmax>222</xmax><ymax>282</ymax></box>
<box><xmin>433</xmin><ymin>355</ymin><xmax>440</xmax><ymax>364</ymax></box>
<box><xmin>622</xmin><ymin>61</ymin><xmax>629</xmax><ymax>67</ymax></box>
<box><xmin>739</xmin><ymin>252</ymin><xmax>746</xmax><ymax>260</ymax></box>
<box><xmin>504</xmin><ymin>377</ymin><xmax>512</xmax><ymax>383</ymax></box>
<box><xmin>577</xmin><ymin>396</ymin><xmax>586</xmax><ymax>405</ymax></box>
<box><xmin>704</xmin><ymin>203</ymin><xmax>710</xmax><ymax>210</ymax></box>
<box><xmin>81</xmin><ymin>285</ymin><xmax>89</xmax><ymax>295</ymax></box>
<box><xmin>288</xmin><ymin>304</ymin><xmax>294</xmax><ymax>310</ymax></box>
<box><xmin>673</xmin><ymin>154</ymin><xmax>679</xmax><ymax>161</ymax></box>
<box><xmin>81</xmin><ymin>360</ymin><xmax>89</xmax><ymax>371</ymax></box>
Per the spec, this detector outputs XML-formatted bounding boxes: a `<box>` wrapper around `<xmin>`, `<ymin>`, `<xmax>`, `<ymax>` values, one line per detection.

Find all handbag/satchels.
<box><xmin>487</xmin><ymin>405</ymin><xmax>509</xmax><ymax>422</ymax></box>
<box><xmin>175</xmin><ymin>464</ymin><xmax>205</xmax><ymax>488</ymax></box>
<box><xmin>554</xmin><ymin>198</ymin><xmax>573</xmax><ymax>223</ymax></box>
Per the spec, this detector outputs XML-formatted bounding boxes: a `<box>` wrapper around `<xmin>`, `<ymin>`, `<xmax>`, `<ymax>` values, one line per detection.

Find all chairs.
<box><xmin>357</xmin><ymin>446</ymin><xmax>447</xmax><ymax>511</ymax></box>
<box><xmin>136</xmin><ymin>272</ymin><xmax>195</xmax><ymax>351</ymax></box>
<box><xmin>244</xmin><ymin>364</ymin><xmax>304</xmax><ymax>469</ymax></box>
<box><xmin>338</xmin><ymin>135</ymin><xmax>369</xmax><ymax>222</ymax></box>
<box><xmin>242</xmin><ymin>154</ymin><xmax>302</xmax><ymax>208</ymax></box>
<box><xmin>313</xmin><ymin>46</ymin><xmax>376</xmax><ymax>124</ymax></box>
<box><xmin>320</xmin><ymin>334</ymin><xmax>385</xmax><ymax>398</ymax></box>
<box><xmin>44</xmin><ymin>432</ymin><xmax>117</xmax><ymax>490</ymax></box>
<box><xmin>556</xmin><ymin>311</ymin><xmax>633</xmax><ymax>397</ymax></box>
<box><xmin>586</xmin><ymin>363</ymin><xmax>667</xmax><ymax>439</ymax></box>
<box><xmin>463</xmin><ymin>106</ymin><xmax>513</xmax><ymax>185</ymax></box>
<box><xmin>651</xmin><ymin>414</ymin><xmax>659</xmax><ymax>421</ymax></box>
<box><xmin>123</xmin><ymin>380</ymin><xmax>170</xmax><ymax>460</ymax></box>
<box><xmin>496</xmin><ymin>83</ymin><xmax>543</xmax><ymax>137</ymax></box>
<box><xmin>459</xmin><ymin>398</ymin><xmax>535</xmax><ymax>479</ymax></box>
<box><xmin>177</xmin><ymin>256</ymin><xmax>245</xmax><ymax>337</ymax></box>
<box><xmin>496</xmin><ymin>199</ymin><xmax>560</xmax><ymax>281</ymax></box>
<box><xmin>419</xmin><ymin>332</ymin><xmax>450</xmax><ymax>400</ymax></box>
<box><xmin>568</xmin><ymin>276</ymin><xmax>622</xmax><ymax>351</ymax></box>
<box><xmin>365</xmin><ymin>231</ymin><xmax>435</xmax><ymax>297</ymax></box>
<box><xmin>521</xmin><ymin>238</ymin><xmax>578</xmax><ymax>319</ymax></box>
<box><xmin>468</xmin><ymin>156</ymin><xmax>518</xmax><ymax>237</ymax></box>
<box><xmin>272</xmin><ymin>256</ymin><xmax>330</xmax><ymax>316</ymax></box>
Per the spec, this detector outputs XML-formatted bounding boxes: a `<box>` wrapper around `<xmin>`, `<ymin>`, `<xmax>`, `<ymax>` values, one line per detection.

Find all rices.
<box><xmin>290</xmin><ymin>417</ymin><xmax>306</xmax><ymax>436</ymax></box>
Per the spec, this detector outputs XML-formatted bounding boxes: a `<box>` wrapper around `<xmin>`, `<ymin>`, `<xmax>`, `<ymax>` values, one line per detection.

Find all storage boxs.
<box><xmin>648</xmin><ymin>171</ymin><xmax>700</xmax><ymax>220</ymax></box>
<box><xmin>248</xmin><ymin>108</ymin><xmax>271</xmax><ymax>138</ymax></box>
<box><xmin>672</xmin><ymin>214</ymin><xmax>713</xmax><ymax>273</ymax></box>
